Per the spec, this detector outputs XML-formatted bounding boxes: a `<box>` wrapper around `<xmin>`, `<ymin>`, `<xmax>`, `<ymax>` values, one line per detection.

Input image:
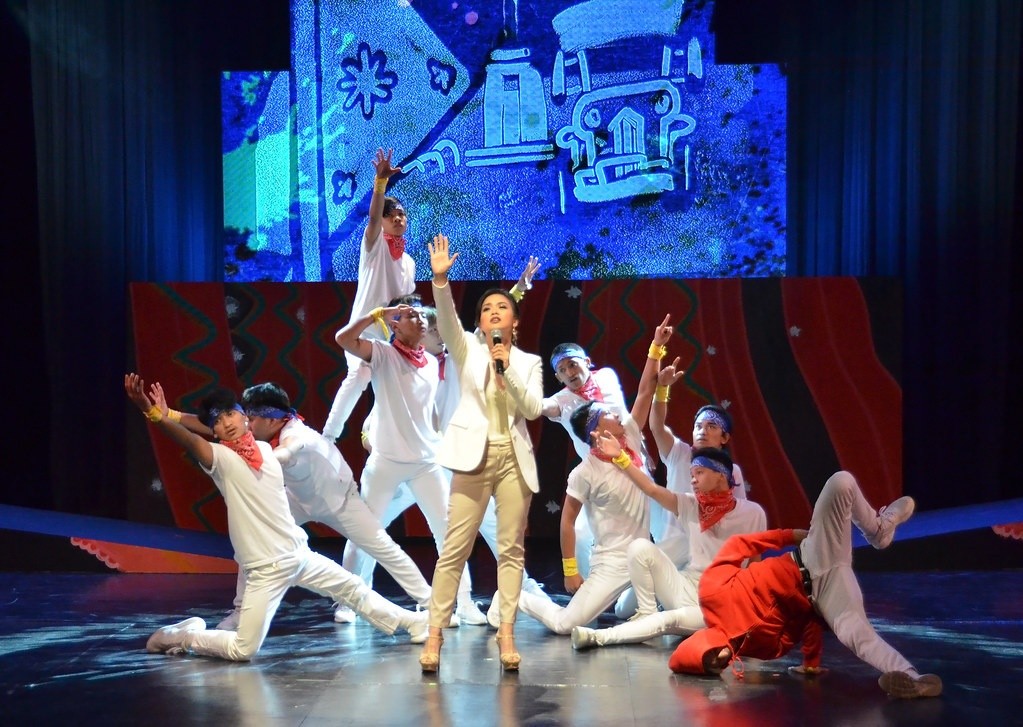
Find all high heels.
<box><xmin>420</xmin><ymin>634</ymin><xmax>445</xmax><ymax>671</ymax></box>
<box><xmin>495</xmin><ymin>628</ymin><xmax>520</xmax><ymax>672</ymax></box>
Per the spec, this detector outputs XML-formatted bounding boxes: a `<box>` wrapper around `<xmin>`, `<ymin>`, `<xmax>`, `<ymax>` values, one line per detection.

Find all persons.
<box><xmin>335</xmin><ymin>297</ymin><xmax>553</xmax><ymax>624</ymax></box>
<box><xmin>125</xmin><ymin>372</ymin><xmax>460</xmax><ymax>660</ymax></box>
<box><xmin>669</xmin><ymin>471</ymin><xmax>943</xmax><ymax>698</ymax></box>
<box><xmin>616</xmin><ymin>357</ymin><xmax>746</xmax><ymax>619</ymax></box>
<box><xmin>570</xmin><ymin>430</ymin><xmax>768</xmax><ymax>650</ymax></box>
<box><xmin>420</xmin><ymin>235</ymin><xmax>543</xmax><ymax>670</ymax></box>
<box><xmin>543</xmin><ymin>343</ymin><xmax>661</xmax><ymax>576</ymax></box>
<box><xmin>487</xmin><ymin>313</ymin><xmax>673</xmax><ymax>634</ymax></box>
<box><xmin>322</xmin><ymin>147</ymin><xmax>416</xmax><ymax>440</ymax></box>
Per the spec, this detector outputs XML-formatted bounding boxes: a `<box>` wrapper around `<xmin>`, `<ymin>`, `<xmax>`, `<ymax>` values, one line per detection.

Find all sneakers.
<box><xmin>571</xmin><ymin>626</ymin><xmax>600</xmax><ymax>649</ymax></box>
<box><xmin>456</xmin><ymin>601</ymin><xmax>488</xmax><ymax>625</ymax></box>
<box><xmin>449</xmin><ymin>613</ymin><xmax>460</xmax><ymax>627</ymax></box>
<box><xmin>872</xmin><ymin>497</ymin><xmax>914</xmax><ymax>550</ymax></box>
<box><xmin>332</xmin><ymin>602</ymin><xmax>355</xmax><ymax>623</ymax></box>
<box><xmin>146</xmin><ymin>617</ymin><xmax>207</xmax><ymax>655</ymax></box>
<box><xmin>878</xmin><ymin>671</ymin><xmax>943</xmax><ymax>698</ymax></box>
<box><xmin>407</xmin><ymin>605</ymin><xmax>430</xmax><ymax>643</ymax></box>
<box><xmin>487</xmin><ymin>590</ymin><xmax>501</xmax><ymax>628</ymax></box>
<box><xmin>216</xmin><ymin>611</ymin><xmax>240</xmax><ymax>632</ymax></box>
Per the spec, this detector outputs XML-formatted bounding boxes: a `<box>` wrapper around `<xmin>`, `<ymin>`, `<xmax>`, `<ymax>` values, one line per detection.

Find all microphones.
<box><xmin>491</xmin><ymin>330</ymin><xmax>504</xmax><ymax>374</ymax></box>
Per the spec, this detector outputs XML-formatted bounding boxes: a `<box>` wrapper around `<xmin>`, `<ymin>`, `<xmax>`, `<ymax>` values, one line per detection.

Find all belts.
<box><xmin>793</xmin><ymin>547</ymin><xmax>812</xmax><ymax>596</ymax></box>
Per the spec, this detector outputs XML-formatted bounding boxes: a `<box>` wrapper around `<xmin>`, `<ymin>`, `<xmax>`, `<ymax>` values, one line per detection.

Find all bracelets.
<box><xmin>612</xmin><ymin>449</ymin><xmax>631</xmax><ymax>470</ymax></box>
<box><xmin>562</xmin><ymin>557</ymin><xmax>578</xmax><ymax>576</ymax></box>
<box><xmin>510</xmin><ymin>284</ymin><xmax>524</xmax><ymax>301</ymax></box>
<box><xmin>144</xmin><ymin>406</ymin><xmax>163</xmax><ymax>422</ymax></box>
<box><xmin>654</xmin><ymin>384</ymin><xmax>669</xmax><ymax>402</ymax></box>
<box><xmin>370</xmin><ymin>306</ymin><xmax>390</xmax><ymax>339</ymax></box>
<box><xmin>374</xmin><ymin>175</ymin><xmax>388</xmax><ymax>193</ymax></box>
<box><xmin>168</xmin><ymin>408</ymin><xmax>182</xmax><ymax>423</ymax></box>
<box><xmin>648</xmin><ymin>341</ymin><xmax>666</xmax><ymax>360</ymax></box>
